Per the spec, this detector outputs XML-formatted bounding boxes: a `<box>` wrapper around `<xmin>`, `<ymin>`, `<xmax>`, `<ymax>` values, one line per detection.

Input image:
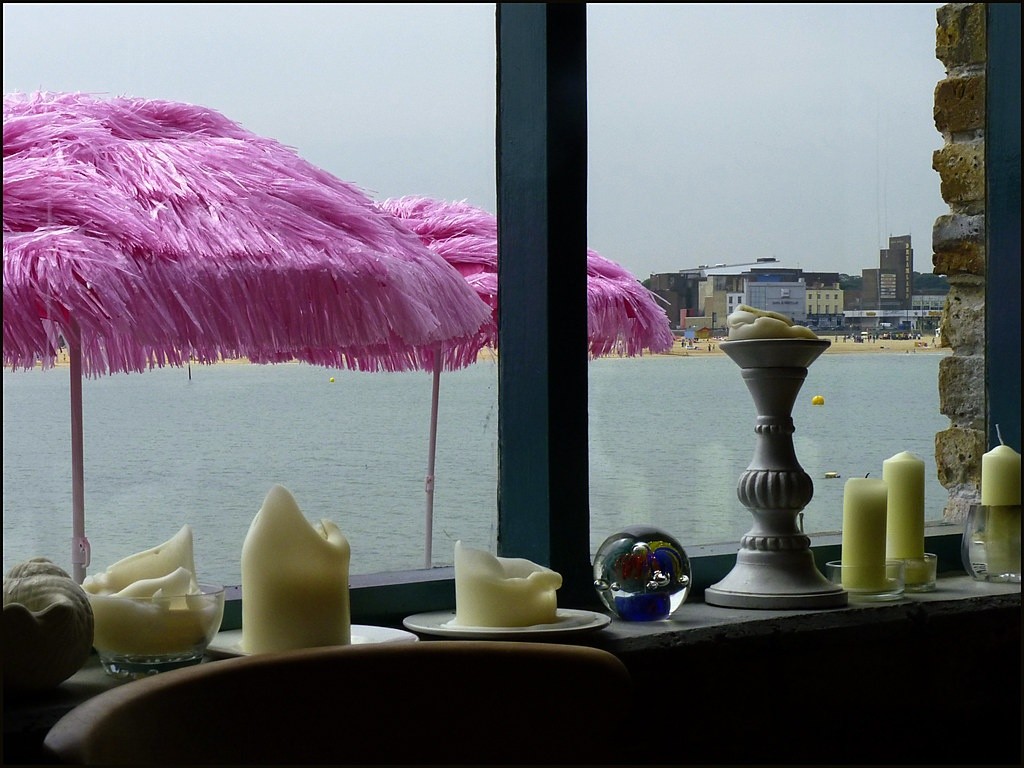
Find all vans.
<box><xmin>860</xmin><ymin>331</ymin><xmax>869</xmax><ymax>339</ymax></box>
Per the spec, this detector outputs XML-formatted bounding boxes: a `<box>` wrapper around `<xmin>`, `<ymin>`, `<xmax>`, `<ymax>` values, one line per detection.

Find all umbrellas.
<box><xmin>371</xmin><ymin>197</ymin><xmax>676</xmax><ymax>572</ymax></box>
<box><xmin>1</xmin><ymin>90</ymin><xmax>494</xmax><ymax>585</ymax></box>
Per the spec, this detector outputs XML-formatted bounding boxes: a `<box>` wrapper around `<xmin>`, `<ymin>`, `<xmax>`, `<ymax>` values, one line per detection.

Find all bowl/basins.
<box><xmin>961</xmin><ymin>505</ymin><xmax>1022</xmax><ymax>582</ymax></box>
<box><xmin>827</xmin><ymin>560</ymin><xmax>905</xmax><ymax>602</ymax></box>
<box><xmin>887</xmin><ymin>553</ymin><xmax>937</xmax><ymax>592</ymax></box>
<box><xmin>85</xmin><ymin>583</ymin><xmax>225</xmax><ymax>679</ymax></box>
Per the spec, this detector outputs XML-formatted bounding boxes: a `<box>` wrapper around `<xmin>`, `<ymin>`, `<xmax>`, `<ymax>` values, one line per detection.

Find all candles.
<box><xmin>978</xmin><ymin>421</ymin><xmax>1021</xmax><ymax>575</ymax></box>
<box><xmin>841</xmin><ymin>473</ymin><xmax>889</xmax><ymax>595</ymax></box>
<box><xmin>239</xmin><ymin>483</ymin><xmax>352</xmax><ymax>655</ymax></box>
<box><xmin>77</xmin><ymin>523</ymin><xmax>217</xmax><ymax>655</ymax></box>
<box><xmin>726</xmin><ymin>301</ymin><xmax>819</xmax><ymax>342</ymax></box>
<box><xmin>453</xmin><ymin>537</ymin><xmax>563</xmax><ymax>627</ymax></box>
<box><xmin>882</xmin><ymin>449</ymin><xmax>926</xmax><ymax>586</ymax></box>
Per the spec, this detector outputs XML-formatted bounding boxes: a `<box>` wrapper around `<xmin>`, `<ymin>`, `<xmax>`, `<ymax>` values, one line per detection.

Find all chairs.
<box><xmin>45</xmin><ymin>642</ymin><xmax>651</xmax><ymax>768</ymax></box>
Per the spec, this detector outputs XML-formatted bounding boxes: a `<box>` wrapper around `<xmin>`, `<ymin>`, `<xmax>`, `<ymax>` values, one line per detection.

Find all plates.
<box><xmin>402</xmin><ymin>608</ymin><xmax>612</xmax><ymax>638</ymax></box>
<box><xmin>205</xmin><ymin>624</ymin><xmax>419</xmax><ymax>658</ymax></box>
